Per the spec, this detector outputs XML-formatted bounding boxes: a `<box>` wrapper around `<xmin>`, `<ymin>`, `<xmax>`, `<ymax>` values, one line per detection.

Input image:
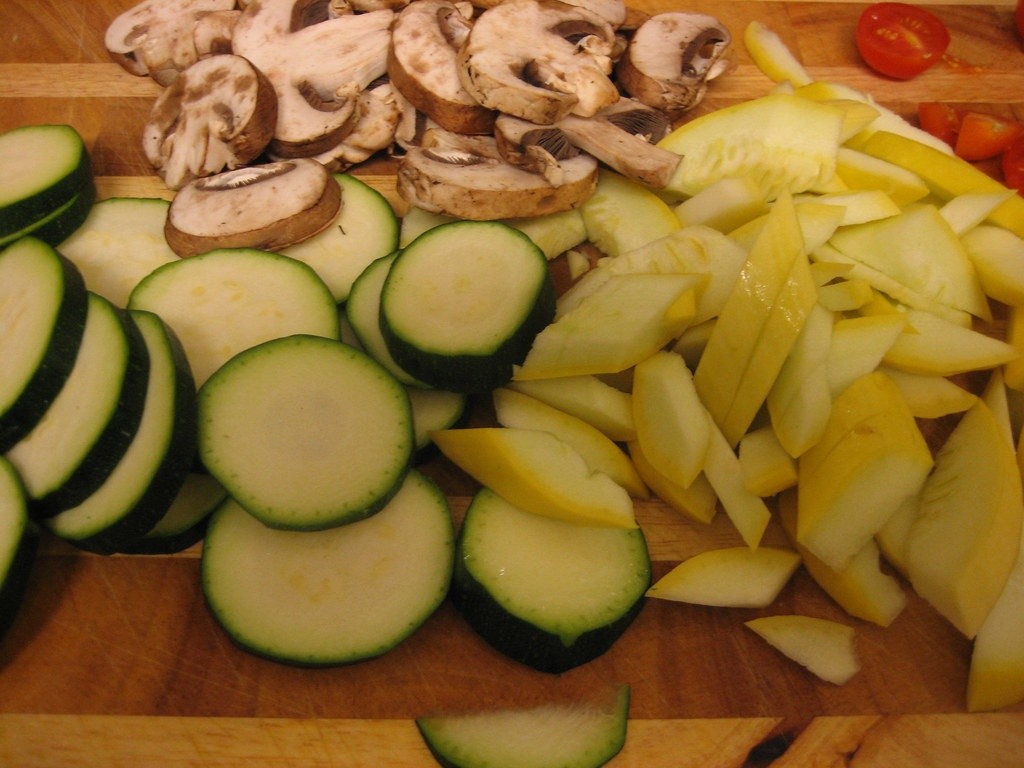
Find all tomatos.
<box><xmin>858</xmin><ymin>3</ymin><xmax>1024</xmax><ymax>200</ymax></box>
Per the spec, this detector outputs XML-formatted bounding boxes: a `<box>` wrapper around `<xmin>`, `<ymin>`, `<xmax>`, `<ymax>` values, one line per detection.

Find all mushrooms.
<box><xmin>104</xmin><ymin>0</ymin><xmax>732</xmax><ymax>259</ymax></box>
